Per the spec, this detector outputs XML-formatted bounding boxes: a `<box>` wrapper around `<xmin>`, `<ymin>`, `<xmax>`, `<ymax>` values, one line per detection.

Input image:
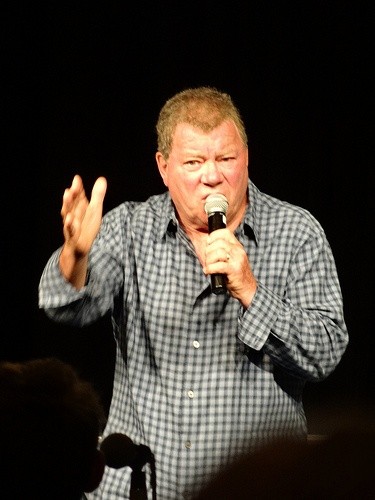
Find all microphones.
<box><xmin>99</xmin><ymin>433</ymin><xmax>155</xmax><ymax>469</ymax></box>
<box><xmin>204</xmin><ymin>193</ymin><xmax>229</xmax><ymax>294</ymax></box>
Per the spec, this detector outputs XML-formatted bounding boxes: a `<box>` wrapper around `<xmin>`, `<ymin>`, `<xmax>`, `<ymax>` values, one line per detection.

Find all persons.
<box><xmin>189</xmin><ymin>427</ymin><xmax>375</xmax><ymax>500</ymax></box>
<box><xmin>39</xmin><ymin>88</ymin><xmax>348</xmax><ymax>500</ymax></box>
<box><xmin>0</xmin><ymin>358</ymin><xmax>107</xmax><ymax>500</ymax></box>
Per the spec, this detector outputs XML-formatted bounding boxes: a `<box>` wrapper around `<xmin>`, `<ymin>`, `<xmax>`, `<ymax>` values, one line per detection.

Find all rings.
<box><xmin>226</xmin><ymin>252</ymin><xmax>230</xmax><ymax>260</ymax></box>
